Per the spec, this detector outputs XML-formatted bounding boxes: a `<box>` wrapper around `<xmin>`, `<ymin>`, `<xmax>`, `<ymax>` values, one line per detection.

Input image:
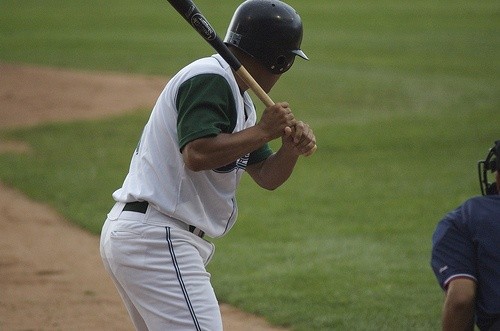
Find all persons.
<box><xmin>100</xmin><ymin>0</ymin><xmax>317</xmax><ymax>331</ymax></box>
<box><xmin>430</xmin><ymin>140</ymin><xmax>500</xmax><ymax>331</ymax></box>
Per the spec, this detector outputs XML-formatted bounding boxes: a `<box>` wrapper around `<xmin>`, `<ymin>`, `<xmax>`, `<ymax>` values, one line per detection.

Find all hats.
<box><xmin>476</xmin><ymin>139</ymin><xmax>500</xmax><ymax>198</ymax></box>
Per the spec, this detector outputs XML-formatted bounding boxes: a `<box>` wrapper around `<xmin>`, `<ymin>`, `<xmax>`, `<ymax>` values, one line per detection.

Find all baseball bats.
<box><xmin>169</xmin><ymin>0</ymin><xmax>298</xmax><ymax>140</ymax></box>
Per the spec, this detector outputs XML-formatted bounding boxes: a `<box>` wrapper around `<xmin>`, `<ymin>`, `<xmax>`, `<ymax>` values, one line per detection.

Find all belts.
<box><xmin>121</xmin><ymin>200</ymin><xmax>149</xmax><ymax>213</ymax></box>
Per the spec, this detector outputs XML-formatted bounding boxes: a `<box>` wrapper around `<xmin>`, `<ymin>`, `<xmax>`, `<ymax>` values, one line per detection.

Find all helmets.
<box><xmin>223</xmin><ymin>0</ymin><xmax>309</xmax><ymax>74</ymax></box>
